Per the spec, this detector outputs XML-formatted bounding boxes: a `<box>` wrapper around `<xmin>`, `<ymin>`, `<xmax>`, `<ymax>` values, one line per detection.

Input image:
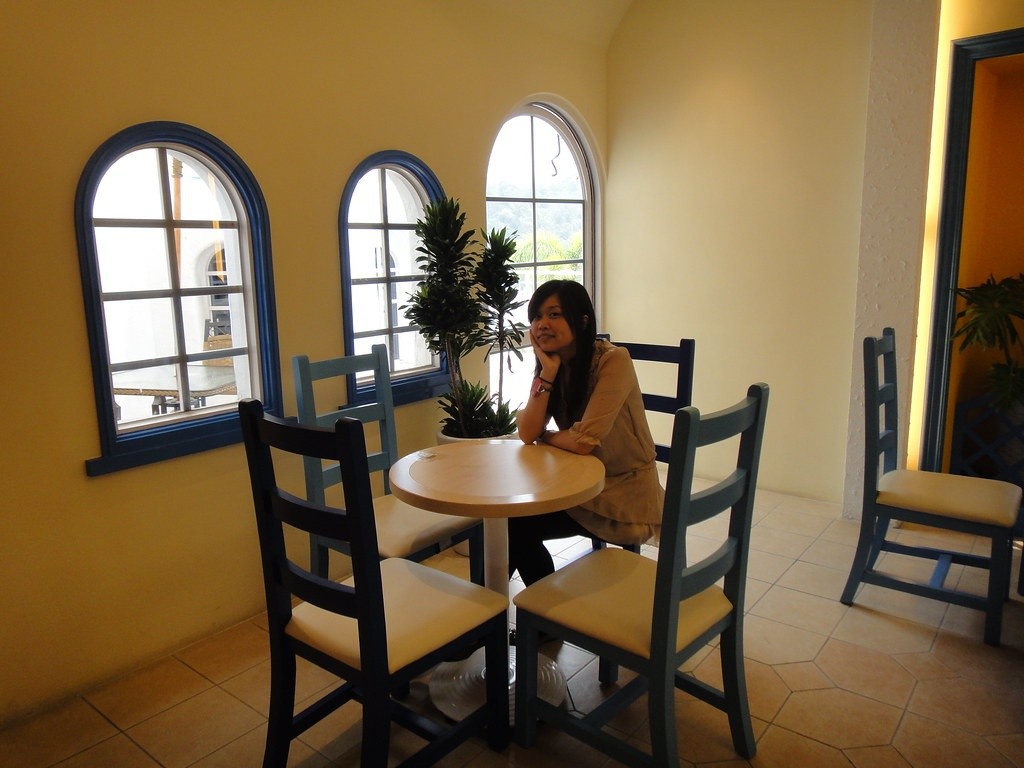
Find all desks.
<box><xmin>112</xmin><ymin>365</ymin><xmax>237</xmax><ymax>413</ymax></box>
<box><xmin>388</xmin><ymin>438</ymin><xmax>606</xmax><ymax>722</ymax></box>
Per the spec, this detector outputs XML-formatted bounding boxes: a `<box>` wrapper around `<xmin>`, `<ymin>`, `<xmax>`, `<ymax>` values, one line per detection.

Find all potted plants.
<box><xmin>398</xmin><ymin>198</ymin><xmax>531</xmax><ymax>557</ymax></box>
<box><xmin>946</xmin><ymin>273</ymin><xmax>1024</xmax><ymax>480</ymax></box>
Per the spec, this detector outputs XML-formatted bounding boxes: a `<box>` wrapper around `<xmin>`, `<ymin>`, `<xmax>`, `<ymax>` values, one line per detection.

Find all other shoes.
<box><xmin>509</xmin><ymin>629</ymin><xmax>517</xmax><ymax>646</ymax></box>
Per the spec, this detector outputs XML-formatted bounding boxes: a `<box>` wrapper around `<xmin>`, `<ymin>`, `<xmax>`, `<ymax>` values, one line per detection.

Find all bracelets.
<box><xmin>532</xmin><ymin>376</ymin><xmax>554</xmax><ymax>397</ymax></box>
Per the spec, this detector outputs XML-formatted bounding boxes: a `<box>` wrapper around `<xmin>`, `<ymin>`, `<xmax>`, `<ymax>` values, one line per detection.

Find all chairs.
<box><xmin>512</xmin><ymin>382</ymin><xmax>771</xmax><ymax>768</ymax></box>
<box><xmin>592</xmin><ymin>333</ymin><xmax>695</xmax><ymax>555</ymax></box>
<box><xmin>200</xmin><ymin>319</ymin><xmax>237</xmax><ymax>406</ymax></box>
<box><xmin>292</xmin><ymin>344</ymin><xmax>485</xmax><ymax>701</ymax></box>
<box><xmin>239</xmin><ymin>398</ymin><xmax>510</xmax><ymax>767</ymax></box>
<box><xmin>839</xmin><ymin>327</ymin><xmax>1023</xmax><ymax>648</ymax></box>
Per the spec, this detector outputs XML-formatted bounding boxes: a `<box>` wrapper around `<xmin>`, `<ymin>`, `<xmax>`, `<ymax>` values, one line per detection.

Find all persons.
<box><xmin>508</xmin><ymin>279</ymin><xmax>667</xmax><ymax>644</ymax></box>
